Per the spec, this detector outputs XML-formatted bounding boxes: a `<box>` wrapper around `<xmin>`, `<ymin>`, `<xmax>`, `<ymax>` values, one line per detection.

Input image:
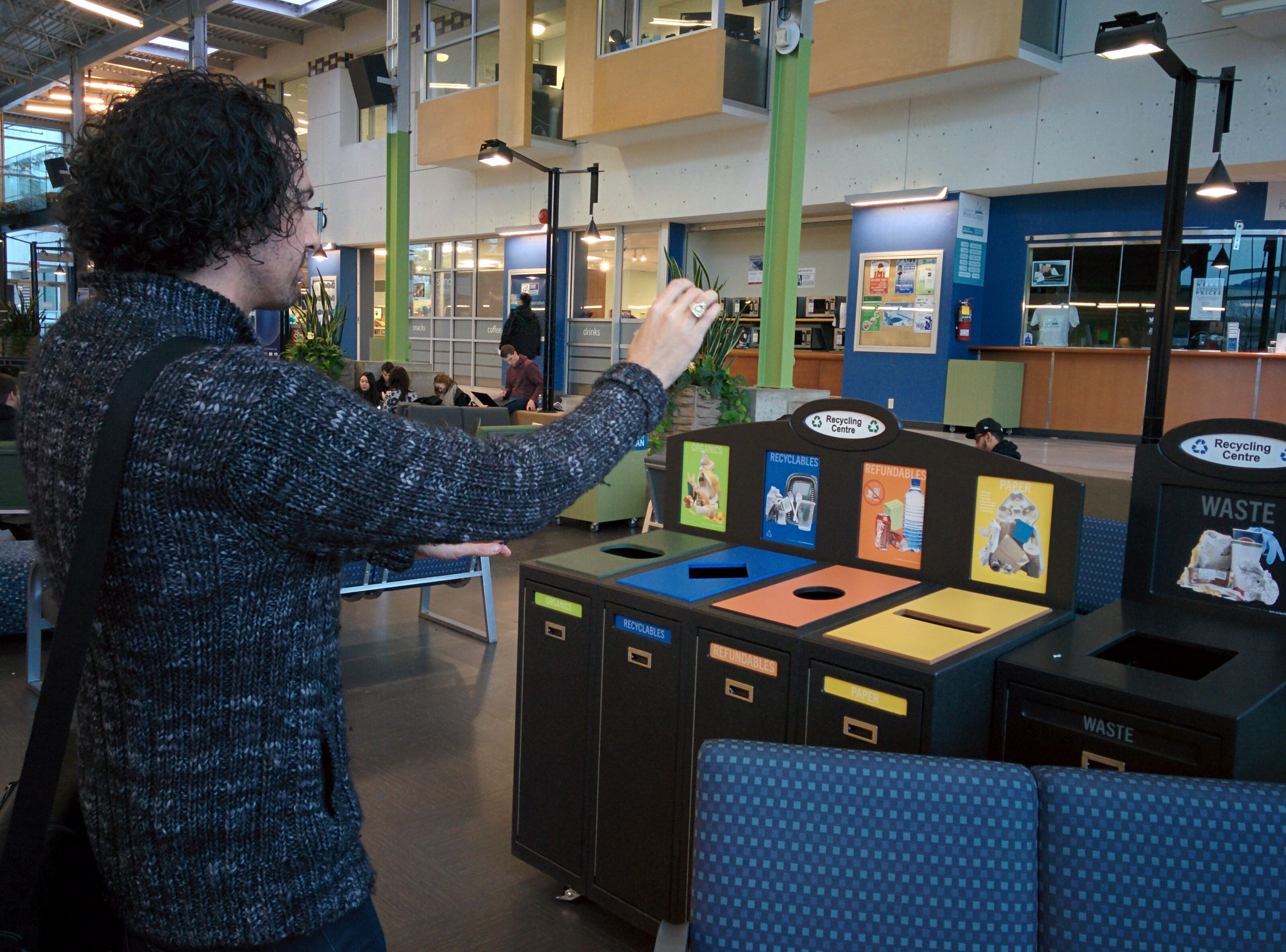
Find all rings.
<box><xmin>690</xmin><ymin>302</ymin><xmax>707</xmax><ymax>318</ymax></box>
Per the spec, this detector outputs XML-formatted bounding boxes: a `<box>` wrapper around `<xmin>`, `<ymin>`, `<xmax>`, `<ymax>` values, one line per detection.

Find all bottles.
<box><xmin>902</xmin><ymin>478</ymin><xmax>925</xmax><ymax>549</ymax></box>
<box><xmin>537</xmin><ymin>394</ymin><xmax>543</xmax><ymax>408</ymax></box>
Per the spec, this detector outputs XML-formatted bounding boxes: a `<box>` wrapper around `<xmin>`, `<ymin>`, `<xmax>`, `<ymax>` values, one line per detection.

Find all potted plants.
<box><xmin>0</xmin><ymin>295</ymin><xmax>52</xmax><ymax>359</ymax></box>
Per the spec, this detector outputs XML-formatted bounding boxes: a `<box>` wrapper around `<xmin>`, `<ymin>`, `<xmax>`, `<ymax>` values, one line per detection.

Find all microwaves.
<box><xmin>794</xmin><ymin>295</ymin><xmax>847</xmax><ymax>351</ymax></box>
<box><xmin>718</xmin><ymin>297</ymin><xmax>760</xmax><ymax>349</ymax></box>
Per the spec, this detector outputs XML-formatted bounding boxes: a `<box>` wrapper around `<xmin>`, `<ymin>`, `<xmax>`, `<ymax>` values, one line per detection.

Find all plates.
<box><xmin>785</xmin><ymin>473</ymin><xmax>817</xmax><ymax>525</ymax></box>
<box><xmin>862</xmin><ymin>303</ymin><xmax>881</xmax><ymax>330</ymax></box>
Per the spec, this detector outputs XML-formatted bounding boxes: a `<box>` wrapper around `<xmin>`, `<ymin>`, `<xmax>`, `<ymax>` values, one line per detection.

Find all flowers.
<box><xmin>635</xmin><ymin>244</ymin><xmax>752</xmax><ymax>453</ymax></box>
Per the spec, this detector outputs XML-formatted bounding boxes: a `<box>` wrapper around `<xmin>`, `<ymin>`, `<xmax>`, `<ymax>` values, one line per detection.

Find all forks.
<box><xmin>787</xmin><ymin>491</ymin><xmax>799</xmax><ymax>523</ymax></box>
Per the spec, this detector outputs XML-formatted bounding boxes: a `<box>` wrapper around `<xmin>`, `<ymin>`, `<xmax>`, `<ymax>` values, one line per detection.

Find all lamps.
<box><xmin>532</xmin><ymin>20</ymin><xmax>546</xmax><ymax>36</ymax></box>
<box><xmin>845</xmin><ymin>186</ymin><xmax>948</xmax><ymax>209</ymax></box>
<box><xmin>55</xmin><ymin>263</ymin><xmax>66</xmax><ymax>275</ymax></box>
<box><xmin>25</xmin><ymin>0</ymin><xmax>222</xmax><ymax>115</ymax></box>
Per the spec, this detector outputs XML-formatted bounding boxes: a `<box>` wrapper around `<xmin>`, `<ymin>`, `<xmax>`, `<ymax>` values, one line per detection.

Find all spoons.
<box><xmin>793</xmin><ymin>492</ymin><xmax>802</xmax><ymax>521</ymax></box>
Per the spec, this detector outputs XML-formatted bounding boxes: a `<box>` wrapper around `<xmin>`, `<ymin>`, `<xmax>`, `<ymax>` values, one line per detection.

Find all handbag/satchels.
<box><xmin>0</xmin><ymin>781</ymin><xmax>133</xmax><ymax>952</ymax></box>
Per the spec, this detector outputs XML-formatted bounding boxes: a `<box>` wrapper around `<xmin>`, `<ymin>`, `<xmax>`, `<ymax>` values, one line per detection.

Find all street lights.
<box><xmin>0</xmin><ymin>233</ymin><xmax>67</xmax><ymax>332</ymax></box>
<box><xmin>475</xmin><ymin>139</ymin><xmax>607</xmax><ymax>409</ymax></box>
<box><xmin>1088</xmin><ymin>11</ymin><xmax>1243</xmax><ymax>598</ymax></box>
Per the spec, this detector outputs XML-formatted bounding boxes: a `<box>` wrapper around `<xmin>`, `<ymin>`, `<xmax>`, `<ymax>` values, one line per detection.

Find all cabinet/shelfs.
<box><xmin>943</xmin><ymin>360</ymin><xmax>1025</xmax><ymax>434</ymax></box>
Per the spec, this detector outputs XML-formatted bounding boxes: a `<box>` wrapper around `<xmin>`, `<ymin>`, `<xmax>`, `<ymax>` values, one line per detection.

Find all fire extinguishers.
<box><xmin>955</xmin><ymin>297</ymin><xmax>974</xmax><ymax>341</ymax></box>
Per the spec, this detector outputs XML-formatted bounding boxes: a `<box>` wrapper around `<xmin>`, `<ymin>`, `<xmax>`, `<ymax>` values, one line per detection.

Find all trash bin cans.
<box><xmin>510</xmin><ymin>399</ymin><xmax>1087</xmax><ymax>940</ymax></box>
<box><xmin>996</xmin><ymin>418</ymin><xmax>1286</xmax><ymax>783</ymax></box>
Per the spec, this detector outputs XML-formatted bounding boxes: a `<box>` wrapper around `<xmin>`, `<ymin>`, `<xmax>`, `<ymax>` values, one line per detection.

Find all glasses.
<box><xmin>974</xmin><ymin>432</ymin><xmax>995</xmax><ymax>444</ymax></box>
<box><xmin>437</xmin><ymin>382</ymin><xmax>443</xmax><ymax>390</ymax></box>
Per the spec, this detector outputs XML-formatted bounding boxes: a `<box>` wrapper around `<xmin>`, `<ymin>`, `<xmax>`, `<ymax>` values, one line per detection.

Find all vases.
<box><xmin>651</xmin><ymin>384</ymin><xmax>719</xmax><ymax>453</ymax></box>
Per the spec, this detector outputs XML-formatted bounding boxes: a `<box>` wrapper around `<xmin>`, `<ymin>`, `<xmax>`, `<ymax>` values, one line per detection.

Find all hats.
<box><xmin>965</xmin><ymin>418</ymin><xmax>1005</xmax><ymax>439</ymax></box>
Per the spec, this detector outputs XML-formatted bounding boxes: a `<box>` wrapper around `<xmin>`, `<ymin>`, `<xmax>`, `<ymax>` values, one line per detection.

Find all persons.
<box><xmin>0</xmin><ymin>373</ymin><xmax>23</xmax><ymax>441</ymax></box>
<box><xmin>376</xmin><ymin>362</ymin><xmax>395</xmax><ymax>400</ymax></box>
<box><xmin>433</xmin><ymin>373</ymin><xmax>471</xmax><ymax>406</ymax></box>
<box><xmin>965</xmin><ymin>417</ymin><xmax>1022</xmax><ymax>461</ymax></box>
<box><xmin>492</xmin><ymin>345</ymin><xmax>554</xmax><ymax>415</ymax></box>
<box><xmin>352</xmin><ymin>372</ymin><xmax>382</xmax><ymax>407</ymax></box>
<box><xmin>497</xmin><ymin>293</ymin><xmax>542</xmax><ymax>360</ymax></box>
<box><xmin>0</xmin><ymin>66</ymin><xmax>721</xmax><ymax>952</ymax></box>
<box><xmin>383</xmin><ymin>366</ymin><xmax>418</xmax><ymax>414</ymax></box>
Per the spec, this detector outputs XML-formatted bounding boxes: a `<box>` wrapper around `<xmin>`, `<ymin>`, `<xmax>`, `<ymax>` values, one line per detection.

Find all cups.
<box><xmin>1268</xmin><ymin>347</ymin><xmax>1276</xmax><ymax>353</ymax></box>
<box><xmin>1232</xmin><ymin>528</ymin><xmax>1264</xmax><ymax>544</ymax></box>
<box><xmin>1023</xmin><ymin>542</ymin><xmax>1040</xmax><ymax>563</ymax></box>
<box><xmin>798</xmin><ymin>500</ymin><xmax>816</xmax><ymax>531</ymax></box>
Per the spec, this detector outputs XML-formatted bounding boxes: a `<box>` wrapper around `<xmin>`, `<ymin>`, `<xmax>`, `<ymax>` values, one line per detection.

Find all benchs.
<box><xmin>26</xmin><ymin>555</ymin><xmax>500</xmax><ymax>694</ymax></box>
<box><xmin>395</xmin><ymin>403</ymin><xmax>546</xmax><ymax>440</ymax></box>
<box><xmin>649</xmin><ymin>735</ymin><xmax>1286</xmax><ymax>951</ymax></box>
